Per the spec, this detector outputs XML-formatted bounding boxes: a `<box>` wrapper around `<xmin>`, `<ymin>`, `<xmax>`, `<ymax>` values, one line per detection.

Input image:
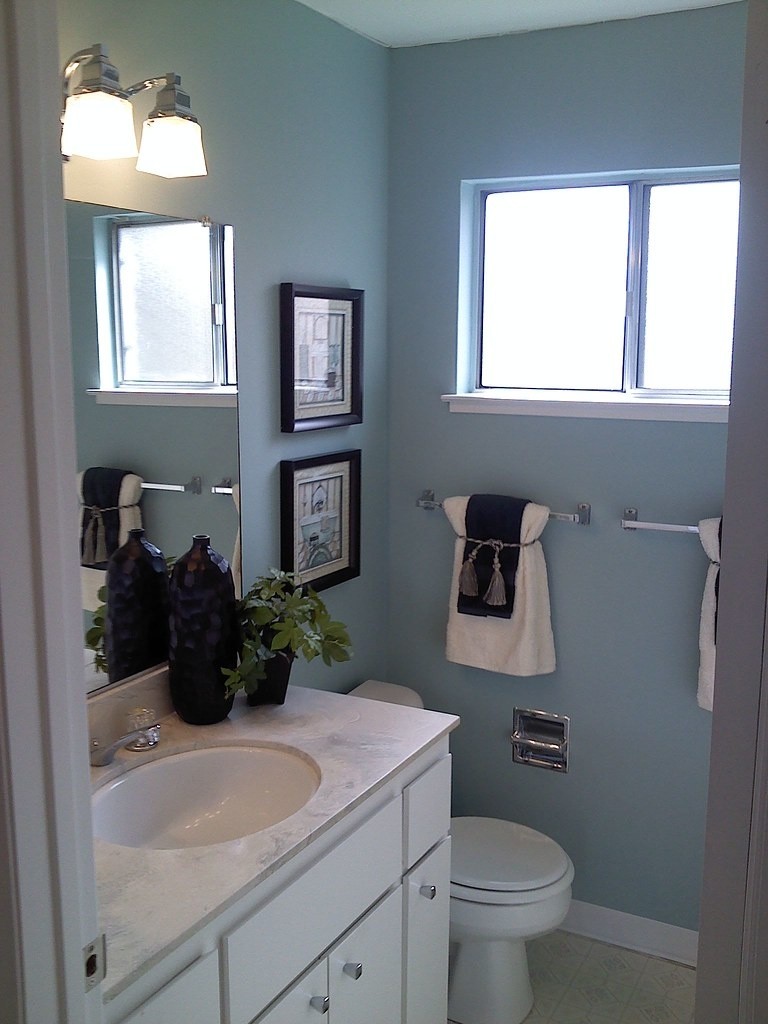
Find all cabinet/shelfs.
<box><xmin>107</xmin><ymin>735</ymin><xmax>452</xmax><ymax>1024</ymax></box>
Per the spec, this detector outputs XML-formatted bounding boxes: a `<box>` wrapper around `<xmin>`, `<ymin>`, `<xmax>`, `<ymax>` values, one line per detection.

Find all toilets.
<box><xmin>346</xmin><ymin>678</ymin><xmax>576</xmax><ymax>1022</ymax></box>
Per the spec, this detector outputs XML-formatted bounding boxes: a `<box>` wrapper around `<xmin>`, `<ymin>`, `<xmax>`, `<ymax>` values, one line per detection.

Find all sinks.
<box><xmin>91</xmin><ymin>737</ymin><xmax>324</xmax><ymax>852</ymax></box>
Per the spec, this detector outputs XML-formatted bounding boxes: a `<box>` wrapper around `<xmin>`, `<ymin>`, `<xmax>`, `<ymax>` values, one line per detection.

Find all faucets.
<box><xmin>89</xmin><ymin>723</ymin><xmax>162</xmax><ymax>767</ymax></box>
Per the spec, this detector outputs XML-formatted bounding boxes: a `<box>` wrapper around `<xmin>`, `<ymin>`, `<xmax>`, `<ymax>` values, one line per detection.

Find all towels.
<box><xmin>229</xmin><ymin>482</ymin><xmax>240</xmax><ymax>577</ymax></box>
<box><xmin>697</xmin><ymin>516</ymin><xmax>723</xmax><ymax>710</ymax></box>
<box><xmin>440</xmin><ymin>494</ymin><xmax>557</xmax><ymax>678</ymax></box>
<box><xmin>75</xmin><ymin>468</ymin><xmax>145</xmax><ymax>613</ymax></box>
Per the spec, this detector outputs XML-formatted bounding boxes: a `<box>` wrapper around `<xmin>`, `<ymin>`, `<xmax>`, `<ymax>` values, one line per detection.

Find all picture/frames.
<box><xmin>279</xmin><ymin>282</ymin><xmax>364</xmax><ymax>433</ymax></box>
<box><xmin>279</xmin><ymin>448</ymin><xmax>362</xmax><ymax>597</ymax></box>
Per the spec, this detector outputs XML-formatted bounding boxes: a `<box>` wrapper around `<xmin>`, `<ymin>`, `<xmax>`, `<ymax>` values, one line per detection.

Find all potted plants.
<box><xmin>222</xmin><ymin>567</ymin><xmax>353</xmax><ymax>707</ymax></box>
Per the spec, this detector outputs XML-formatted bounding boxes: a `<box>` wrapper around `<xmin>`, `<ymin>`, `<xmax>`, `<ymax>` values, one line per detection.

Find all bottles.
<box><xmin>168</xmin><ymin>533</ymin><xmax>236</xmax><ymax>725</ymax></box>
<box><xmin>106</xmin><ymin>528</ymin><xmax>168</xmax><ymax>682</ymax></box>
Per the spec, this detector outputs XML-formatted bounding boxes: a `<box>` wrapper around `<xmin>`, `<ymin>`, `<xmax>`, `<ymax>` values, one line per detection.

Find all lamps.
<box><xmin>58</xmin><ymin>43</ymin><xmax>209</xmax><ymax>179</ymax></box>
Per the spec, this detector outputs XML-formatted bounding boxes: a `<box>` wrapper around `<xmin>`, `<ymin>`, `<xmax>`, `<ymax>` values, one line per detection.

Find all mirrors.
<box><xmin>64</xmin><ymin>199</ymin><xmax>243</xmax><ymax>692</ymax></box>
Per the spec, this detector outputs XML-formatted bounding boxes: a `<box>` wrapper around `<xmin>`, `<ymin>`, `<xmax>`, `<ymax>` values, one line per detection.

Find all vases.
<box><xmin>168</xmin><ymin>533</ymin><xmax>238</xmax><ymax>727</ymax></box>
<box><xmin>105</xmin><ymin>528</ymin><xmax>172</xmax><ymax>681</ymax></box>
